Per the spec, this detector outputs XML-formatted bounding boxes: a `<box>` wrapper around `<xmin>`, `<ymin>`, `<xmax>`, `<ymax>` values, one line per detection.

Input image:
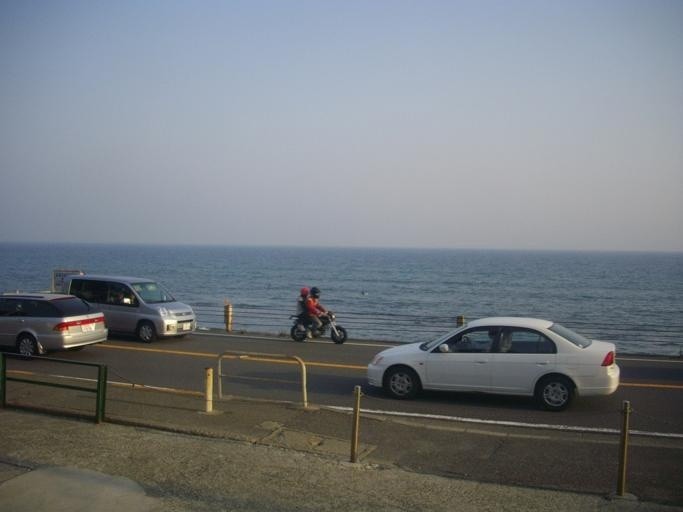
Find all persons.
<box><xmin>303</xmin><ymin>286</ymin><xmax>328</xmax><ymax>338</ymax></box>
<box><xmin>295</xmin><ymin>286</ymin><xmax>309</xmax><ymax>332</ymax></box>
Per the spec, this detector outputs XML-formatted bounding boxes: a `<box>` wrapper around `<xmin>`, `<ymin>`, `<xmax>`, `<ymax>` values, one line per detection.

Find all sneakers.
<box><xmin>296</xmin><ymin>324</ymin><xmax>305</xmax><ymax>331</ymax></box>
<box><xmin>306</xmin><ymin>330</ymin><xmax>312</xmax><ymax>339</ymax></box>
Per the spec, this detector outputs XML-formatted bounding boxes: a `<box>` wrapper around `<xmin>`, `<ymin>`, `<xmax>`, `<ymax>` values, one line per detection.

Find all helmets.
<box><xmin>310</xmin><ymin>287</ymin><xmax>319</xmax><ymax>298</ymax></box>
<box><xmin>301</xmin><ymin>288</ymin><xmax>308</xmax><ymax>297</ymax></box>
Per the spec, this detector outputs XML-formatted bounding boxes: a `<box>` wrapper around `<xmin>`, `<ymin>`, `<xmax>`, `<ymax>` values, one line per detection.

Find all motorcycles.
<box><xmin>288</xmin><ymin>311</ymin><xmax>348</xmax><ymax>344</ymax></box>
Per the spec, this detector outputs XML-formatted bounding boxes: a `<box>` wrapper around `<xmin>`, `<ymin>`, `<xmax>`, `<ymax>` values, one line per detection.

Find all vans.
<box><xmin>61</xmin><ymin>274</ymin><xmax>196</xmax><ymax>343</ymax></box>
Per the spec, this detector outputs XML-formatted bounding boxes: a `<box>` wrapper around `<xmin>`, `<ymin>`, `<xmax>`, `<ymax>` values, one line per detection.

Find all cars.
<box><xmin>0</xmin><ymin>293</ymin><xmax>109</xmax><ymax>361</ymax></box>
<box><xmin>367</xmin><ymin>316</ymin><xmax>622</xmax><ymax>411</ymax></box>
